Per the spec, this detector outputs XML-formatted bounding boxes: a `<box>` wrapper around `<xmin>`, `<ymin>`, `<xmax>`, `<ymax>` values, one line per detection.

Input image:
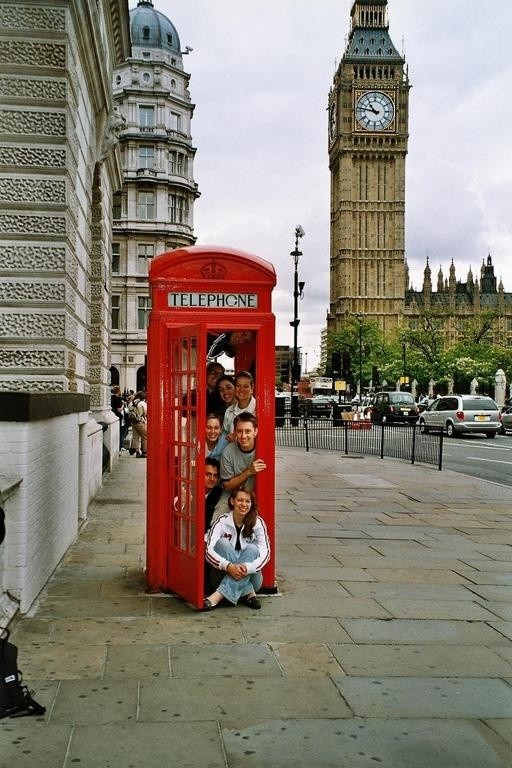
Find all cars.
<box><xmin>364</xmin><ymin>391</ymin><xmax>512</xmax><ymax>437</ymax></box>
<box><xmin>298</xmin><ymin>395</ymin><xmax>351</xmax><ymax>418</ymax></box>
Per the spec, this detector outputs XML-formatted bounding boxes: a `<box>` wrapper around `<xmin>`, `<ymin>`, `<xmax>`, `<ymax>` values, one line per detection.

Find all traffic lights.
<box><xmin>281</xmin><ymin>360</ymin><xmax>289</xmax><ymax>384</ymax></box>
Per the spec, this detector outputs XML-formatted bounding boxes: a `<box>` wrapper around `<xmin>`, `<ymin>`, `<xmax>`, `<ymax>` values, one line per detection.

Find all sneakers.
<box><xmin>135</xmin><ymin>451</ymin><xmax>147</xmax><ymax>458</ymax></box>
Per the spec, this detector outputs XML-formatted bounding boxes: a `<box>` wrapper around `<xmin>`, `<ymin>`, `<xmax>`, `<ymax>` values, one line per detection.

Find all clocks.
<box><xmin>354</xmin><ymin>90</ymin><xmax>396</xmax><ymax>132</ymax></box>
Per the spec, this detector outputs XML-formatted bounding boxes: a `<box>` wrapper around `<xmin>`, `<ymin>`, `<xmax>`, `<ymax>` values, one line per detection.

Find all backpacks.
<box><xmin>127</xmin><ymin>400</ymin><xmax>146</xmax><ymax>424</ymax></box>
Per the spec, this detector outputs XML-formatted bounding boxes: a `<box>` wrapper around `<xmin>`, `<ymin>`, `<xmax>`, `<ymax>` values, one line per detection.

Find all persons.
<box><xmin>174</xmin><ymin>328</ymin><xmax>273</xmax><ymax>610</ymax></box>
<box><xmin>417</xmin><ymin>392</ymin><xmax>442</xmax><ymax>403</ymax></box>
<box><xmin>109</xmin><ymin>384</ymin><xmax>148</xmax><ymax>458</ymax></box>
<box><xmin>344</xmin><ymin>389</ymin><xmax>376</xmax><ymax>405</ymax></box>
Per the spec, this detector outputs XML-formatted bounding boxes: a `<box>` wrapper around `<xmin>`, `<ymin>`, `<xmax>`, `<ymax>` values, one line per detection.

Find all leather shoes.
<box><xmin>245</xmin><ymin>596</ymin><xmax>262</xmax><ymax>610</ymax></box>
<box><xmin>197</xmin><ymin>599</ymin><xmax>219</xmax><ymax>612</ymax></box>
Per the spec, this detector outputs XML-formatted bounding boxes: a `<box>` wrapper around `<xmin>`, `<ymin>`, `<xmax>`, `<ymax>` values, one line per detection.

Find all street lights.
<box><xmin>356</xmin><ymin>312</ymin><xmax>364</xmax><ymax>393</ymax></box>
<box><xmin>290</xmin><ymin>225</ymin><xmax>305</xmax><ymax>426</ymax></box>
<box><xmin>399</xmin><ymin>334</ymin><xmax>406</xmax><ymax>376</ymax></box>
